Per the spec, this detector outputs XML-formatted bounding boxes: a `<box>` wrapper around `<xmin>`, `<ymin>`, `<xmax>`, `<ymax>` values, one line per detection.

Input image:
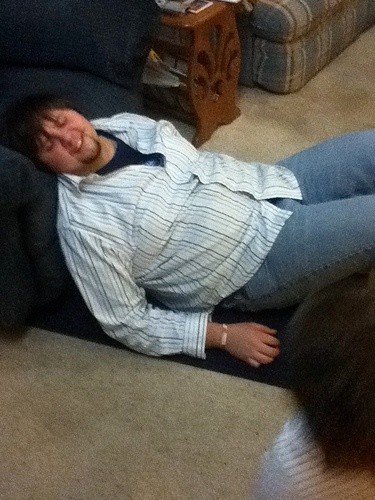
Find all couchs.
<box><xmin>1</xmin><ymin>0</ymin><xmax>162</xmax><ymax>337</ymax></box>
<box><xmin>232</xmin><ymin>0</ymin><xmax>375</xmax><ymax>98</ymax></box>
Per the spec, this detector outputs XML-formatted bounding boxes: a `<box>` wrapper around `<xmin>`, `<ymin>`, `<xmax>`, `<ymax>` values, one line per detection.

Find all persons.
<box><xmin>254</xmin><ymin>270</ymin><xmax>375</xmax><ymax>499</ymax></box>
<box><xmin>1</xmin><ymin>93</ymin><xmax>375</xmax><ymax>368</ymax></box>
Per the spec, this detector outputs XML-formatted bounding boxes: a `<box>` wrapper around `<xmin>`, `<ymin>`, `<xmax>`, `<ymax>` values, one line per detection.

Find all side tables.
<box><xmin>137</xmin><ymin>1</ymin><xmax>245</xmax><ymax>150</ymax></box>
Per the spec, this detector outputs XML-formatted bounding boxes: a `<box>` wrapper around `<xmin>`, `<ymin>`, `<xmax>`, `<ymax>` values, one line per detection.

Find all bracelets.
<box><xmin>221</xmin><ymin>322</ymin><xmax>228</xmax><ymax>353</ymax></box>
